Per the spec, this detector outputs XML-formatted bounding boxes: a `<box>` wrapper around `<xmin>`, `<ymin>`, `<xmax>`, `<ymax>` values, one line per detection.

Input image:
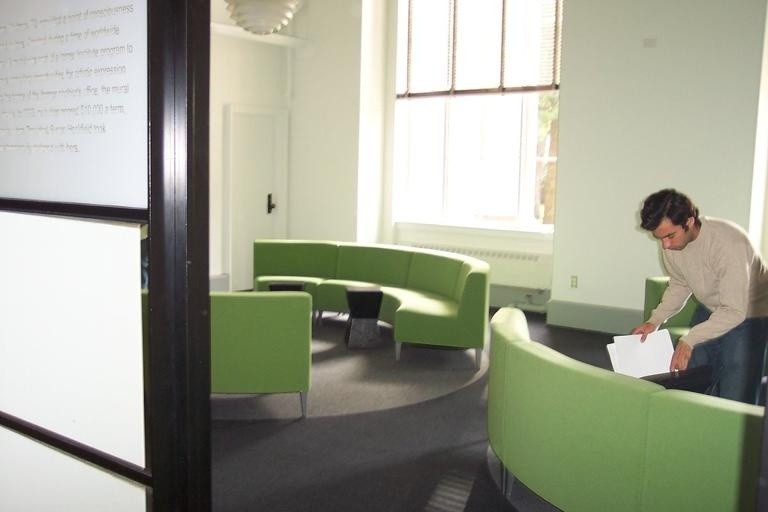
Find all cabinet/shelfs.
<box><xmin>225</xmin><ymin>107</ymin><xmax>289</xmax><ymax>293</ymax></box>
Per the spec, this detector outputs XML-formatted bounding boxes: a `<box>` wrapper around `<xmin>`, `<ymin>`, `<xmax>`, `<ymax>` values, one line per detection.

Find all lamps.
<box><xmin>222</xmin><ymin>1</ymin><xmax>302</xmax><ymax>36</ymax></box>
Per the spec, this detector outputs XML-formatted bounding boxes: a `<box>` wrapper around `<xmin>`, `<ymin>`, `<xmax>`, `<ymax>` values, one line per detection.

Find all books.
<box><xmin>605</xmin><ymin>325</ymin><xmax>715</xmax><ymax>394</ymax></box>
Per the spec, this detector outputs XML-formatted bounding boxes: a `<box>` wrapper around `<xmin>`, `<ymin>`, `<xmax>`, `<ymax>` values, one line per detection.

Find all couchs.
<box><xmin>251</xmin><ymin>240</ymin><xmax>490</xmax><ymax>370</ymax></box>
<box><xmin>205</xmin><ymin>288</ymin><xmax>312</xmax><ymax>418</ymax></box>
<box><xmin>484</xmin><ymin>305</ymin><xmax>767</xmax><ymax>512</ymax></box>
<box><xmin>643</xmin><ymin>275</ymin><xmax>699</xmax><ymax>350</ymax></box>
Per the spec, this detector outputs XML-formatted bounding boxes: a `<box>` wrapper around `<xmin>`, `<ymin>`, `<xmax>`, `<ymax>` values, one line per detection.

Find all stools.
<box><xmin>267</xmin><ymin>282</ymin><xmax>382</xmax><ymax>349</ymax></box>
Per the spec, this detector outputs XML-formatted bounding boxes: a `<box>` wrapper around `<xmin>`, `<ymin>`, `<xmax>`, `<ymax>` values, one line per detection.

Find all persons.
<box><xmin>626</xmin><ymin>188</ymin><xmax>767</xmax><ymax>405</ymax></box>
